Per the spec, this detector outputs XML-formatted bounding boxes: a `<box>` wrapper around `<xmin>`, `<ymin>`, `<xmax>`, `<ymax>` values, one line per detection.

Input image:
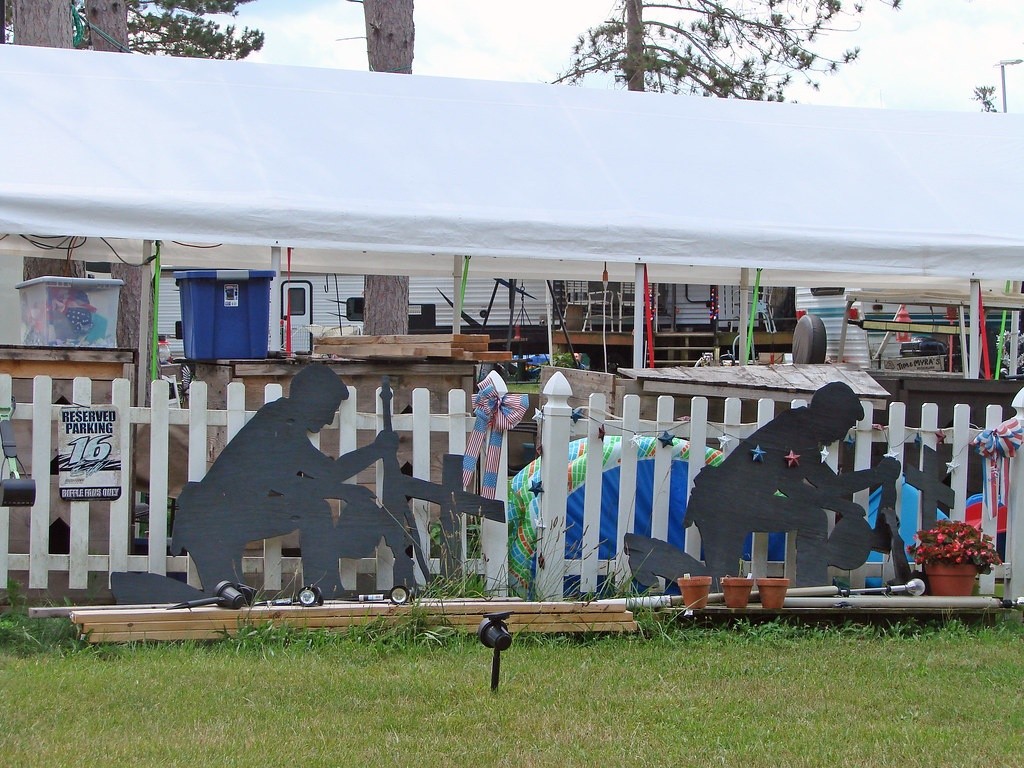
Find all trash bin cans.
<box><xmin>172</xmin><ymin>269</ymin><xmax>277</xmax><ymax>361</ymax></box>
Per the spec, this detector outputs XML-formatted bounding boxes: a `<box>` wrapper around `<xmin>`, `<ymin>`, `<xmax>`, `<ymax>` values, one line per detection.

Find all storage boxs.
<box><xmin>15</xmin><ymin>276</ymin><xmax>124</xmax><ymax>349</ymax></box>
<box><xmin>884</xmin><ymin>356</ymin><xmax>944</xmax><ymax>372</ymax></box>
<box><xmin>172</xmin><ymin>271</ymin><xmax>277</xmax><ymax>359</ymax></box>
<box><xmin>901</xmin><ymin>341</ymin><xmax>922</xmax><ymax>357</ymax></box>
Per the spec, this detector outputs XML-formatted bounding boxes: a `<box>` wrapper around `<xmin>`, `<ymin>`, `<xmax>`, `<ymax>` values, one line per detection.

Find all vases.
<box><xmin>756</xmin><ymin>578</ymin><xmax>790</xmax><ymax>608</ymax></box>
<box><xmin>720</xmin><ymin>577</ymin><xmax>754</xmax><ymax>608</ymax></box>
<box><xmin>921</xmin><ymin>557</ymin><xmax>977</xmax><ymax>596</ymax></box>
<box><xmin>677</xmin><ymin>577</ymin><xmax>712</xmax><ymax>609</ymax></box>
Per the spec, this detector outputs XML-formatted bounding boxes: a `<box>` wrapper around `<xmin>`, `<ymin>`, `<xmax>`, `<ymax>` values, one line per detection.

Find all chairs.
<box><xmin>561</xmin><ymin>279</ymin><xmax>614</xmax><ymax>332</ymax></box>
<box><xmin>729</xmin><ymin>286</ymin><xmax>777</xmax><ymax>334</ymax></box>
<box><xmin>617</xmin><ymin>282</ymin><xmax>660</xmax><ymax>334</ymax></box>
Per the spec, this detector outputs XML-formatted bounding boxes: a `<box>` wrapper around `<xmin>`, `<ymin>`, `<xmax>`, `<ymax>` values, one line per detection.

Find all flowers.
<box><xmin>906</xmin><ymin>518</ymin><xmax>1001</xmax><ymax>575</ymax></box>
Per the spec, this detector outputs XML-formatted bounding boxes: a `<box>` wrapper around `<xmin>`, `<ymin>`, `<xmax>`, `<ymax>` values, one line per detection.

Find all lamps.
<box><xmin>477</xmin><ymin>610</ymin><xmax>514</xmax><ymax>692</ymax></box>
<box><xmin>169</xmin><ymin>580</ymin><xmax>243</xmax><ymax>609</ymax></box>
<box><xmin>337</xmin><ymin>586</ymin><xmax>409</xmax><ymax>605</ymax></box>
<box><xmin>256</xmin><ymin>585</ymin><xmax>323</xmax><ymax>606</ymax></box>
